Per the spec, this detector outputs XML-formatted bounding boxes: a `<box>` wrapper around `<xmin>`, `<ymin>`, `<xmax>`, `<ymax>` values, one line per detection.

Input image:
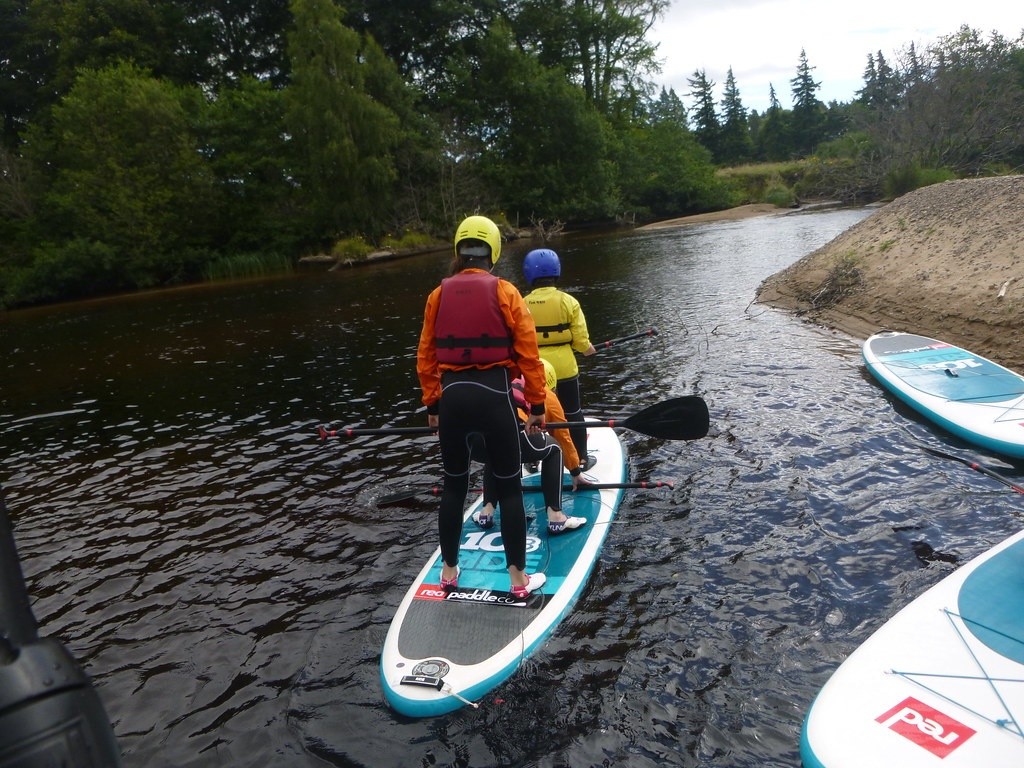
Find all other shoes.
<box><xmin>472</xmin><ymin>511</ymin><xmax>495</xmax><ymax>530</ymax></box>
<box><xmin>525</xmin><ymin>461</ymin><xmax>540</xmax><ymax>473</ymax></box>
<box><xmin>580</xmin><ymin>455</ymin><xmax>597</xmax><ymax>471</ymax></box>
<box><xmin>546</xmin><ymin>515</ymin><xmax>587</xmax><ymax>533</ymax></box>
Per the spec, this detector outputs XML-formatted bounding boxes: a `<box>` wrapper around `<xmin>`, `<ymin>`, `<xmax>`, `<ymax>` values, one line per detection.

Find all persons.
<box><xmin>473</xmin><ymin>358</ymin><xmax>587</xmax><ymax>535</ymax></box>
<box><xmin>417</xmin><ymin>216</ymin><xmax>547</xmax><ymax>600</ymax></box>
<box><xmin>520</xmin><ymin>248</ymin><xmax>599</xmax><ymax>473</ymax></box>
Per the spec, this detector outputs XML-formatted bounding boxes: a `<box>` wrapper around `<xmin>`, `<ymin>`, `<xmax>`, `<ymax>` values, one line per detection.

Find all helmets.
<box><xmin>454</xmin><ymin>215</ymin><xmax>502</xmax><ymax>264</ymax></box>
<box><xmin>523</xmin><ymin>248</ymin><xmax>561</xmax><ymax>284</ymax></box>
<box><xmin>539</xmin><ymin>357</ymin><xmax>557</xmax><ymax>392</ymax></box>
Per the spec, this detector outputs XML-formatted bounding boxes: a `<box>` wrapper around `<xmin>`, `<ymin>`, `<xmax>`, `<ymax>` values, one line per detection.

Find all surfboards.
<box><xmin>802</xmin><ymin>529</ymin><xmax>1024</xmax><ymax>768</ymax></box>
<box><xmin>379</xmin><ymin>410</ymin><xmax>629</xmax><ymax>718</ymax></box>
<box><xmin>864</xmin><ymin>329</ymin><xmax>1024</xmax><ymax>458</ymax></box>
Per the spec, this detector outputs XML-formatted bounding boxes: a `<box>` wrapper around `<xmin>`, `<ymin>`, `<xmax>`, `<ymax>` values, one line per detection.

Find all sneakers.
<box><xmin>440</xmin><ymin>565</ymin><xmax>460</xmax><ymax>592</ymax></box>
<box><xmin>510</xmin><ymin>572</ymin><xmax>546</xmax><ymax>602</ymax></box>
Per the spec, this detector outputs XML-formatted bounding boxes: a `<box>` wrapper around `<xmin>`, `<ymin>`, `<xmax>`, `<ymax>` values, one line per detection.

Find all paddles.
<box><xmin>376</xmin><ymin>476</ymin><xmax>677</xmax><ymax>506</ymax></box>
<box><xmin>914</xmin><ymin>443</ymin><xmax>1024</xmax><ymax>494</ymax></box>
<box><xmin>320</xmin><ymin>396</ymin><xmax>710</xmax><ymax>445</ymax></box>
<box><xmin>574</xmin><ymin>326</ymin><xmax>659</xmax><ymax>356</ymax></box>
